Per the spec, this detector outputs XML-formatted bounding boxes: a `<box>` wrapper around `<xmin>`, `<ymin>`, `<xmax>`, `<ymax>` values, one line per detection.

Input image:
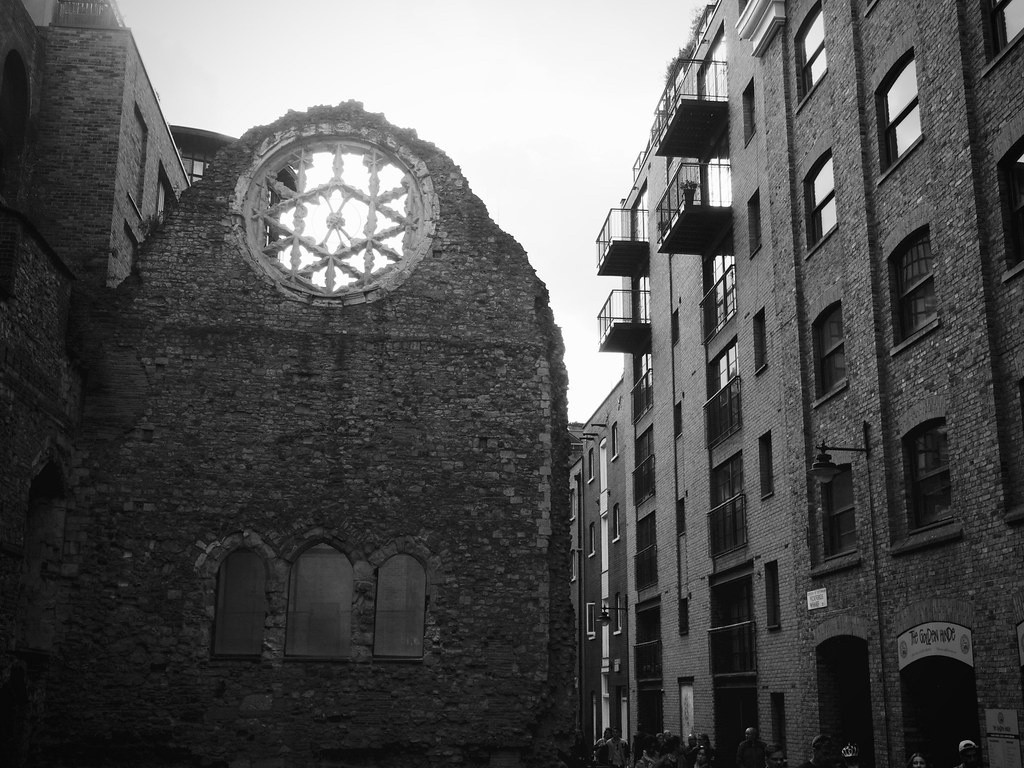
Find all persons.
<box><xmin>797</xmin><ymin>734</ymin><xmax>860</xmax><ymax>768</ymax></box>
<box><xmin>632</xmin><ymin>722</ymin><xmax>715</xmax><ymax>768</ymax></box>
<box><xmin>596</xmin><ymin>726</ymin><xmax>629</xmax><ymax>768</ymax></box>
<box><xmin>736</xmin><ymin>727</ymin><xmax>784</xmax><ymax>768</ymax></box>
<box><xmin>953</xmin><ymin>740</ymin><xmax>979</xmax><ymax>768</ymax></box>
<box><xmin>906</xmin><ymin>752</ymin><xmax>931</xmax><ymax>768</ymax></box>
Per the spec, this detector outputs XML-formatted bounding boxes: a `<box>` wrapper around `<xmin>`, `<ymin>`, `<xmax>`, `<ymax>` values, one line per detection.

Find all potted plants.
<box><xmin>679</xmin><ymin>179</ymin><xmax>698</xmax><ymax>205</ymax></box>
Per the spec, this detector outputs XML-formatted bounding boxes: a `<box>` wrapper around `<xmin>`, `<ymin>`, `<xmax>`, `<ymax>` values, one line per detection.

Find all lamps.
<box><xmin>595</xmin><ymin>603</ymin><xmax>627</xmax><ymax>626</ymax></box>
<box><xmin>810</xmin><ymin>419</ymin><xmax>870</xmax><ymax>484</ymax></box>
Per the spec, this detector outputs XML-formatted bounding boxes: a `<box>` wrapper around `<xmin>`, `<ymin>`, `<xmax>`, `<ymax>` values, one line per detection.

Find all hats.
<box><xmin>812</xmin><ymin>734</ymin><xmax>836</xmax><ymax>749</ymax></box>
<box><xmin>841</xmin><ymin>742</ymin><xmax>861</xmax><ymax>759</ymax></box>
<box><xmin>958</xmin><ymin>739</ymin><xmax>979</xmax><ymax>752</ymax></box>
